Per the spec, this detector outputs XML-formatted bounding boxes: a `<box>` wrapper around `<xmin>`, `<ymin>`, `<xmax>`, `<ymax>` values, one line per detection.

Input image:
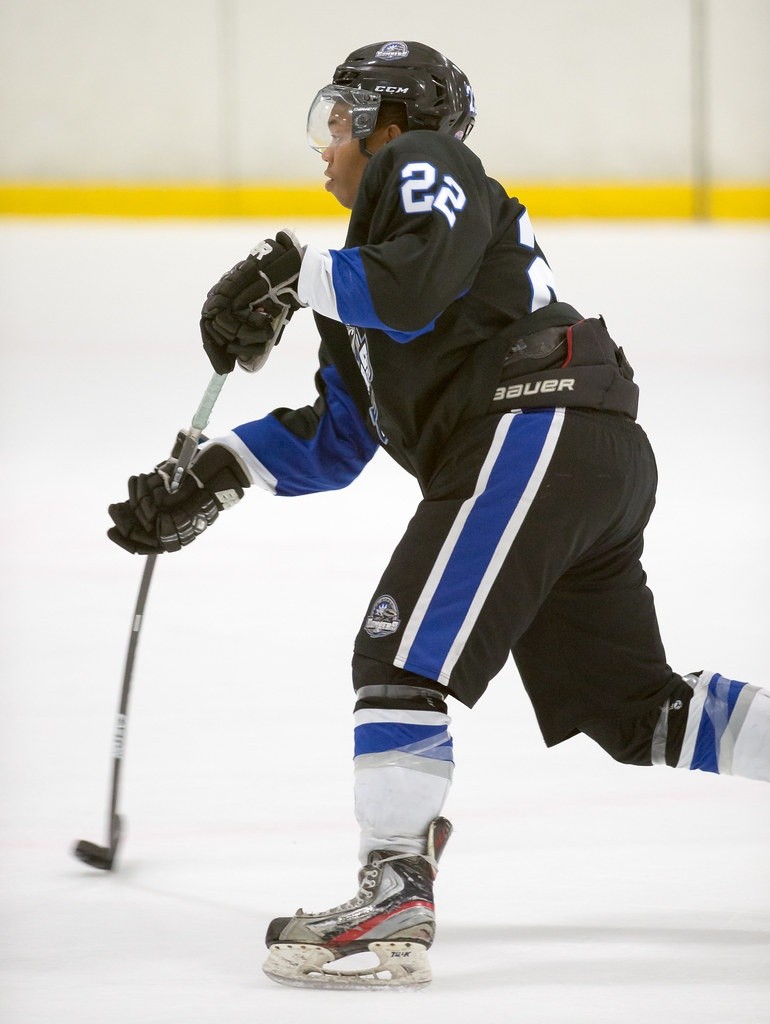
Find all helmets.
<box><xmin>305</xmin><ymin>40</ymin><xmax>477</xmax><ymax>154</ymax></box>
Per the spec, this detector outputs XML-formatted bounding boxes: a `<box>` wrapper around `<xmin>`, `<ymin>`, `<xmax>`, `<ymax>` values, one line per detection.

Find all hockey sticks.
<box><xmin>72</xmin><ymin>312</ymin><xmax>267</xmax><ymax>871</ymax></box>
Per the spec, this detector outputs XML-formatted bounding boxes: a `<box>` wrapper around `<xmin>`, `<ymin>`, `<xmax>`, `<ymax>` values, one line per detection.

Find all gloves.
<box><xmin>198</xmin><ymin>228</ymin><xmax>309</xmax><ymax>375</ymax></box>
<box><xmin>104</xmin><ymin>428</ymin><xmax>255</xmax><ymax>555</ymax></box>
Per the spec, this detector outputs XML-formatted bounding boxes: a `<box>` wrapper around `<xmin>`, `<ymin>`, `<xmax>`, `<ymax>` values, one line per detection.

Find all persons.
<box><xmin>106</xmin><ymin>41</ymin><xmax>770</xmax><ymax>991</ymax></box>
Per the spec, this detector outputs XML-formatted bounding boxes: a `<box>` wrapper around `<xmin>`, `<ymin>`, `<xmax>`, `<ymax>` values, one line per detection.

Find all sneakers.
<box><xmin>260</xmin><ymin>813</ymin><xmax>455</xmax><ymax>992</ymax></box>
